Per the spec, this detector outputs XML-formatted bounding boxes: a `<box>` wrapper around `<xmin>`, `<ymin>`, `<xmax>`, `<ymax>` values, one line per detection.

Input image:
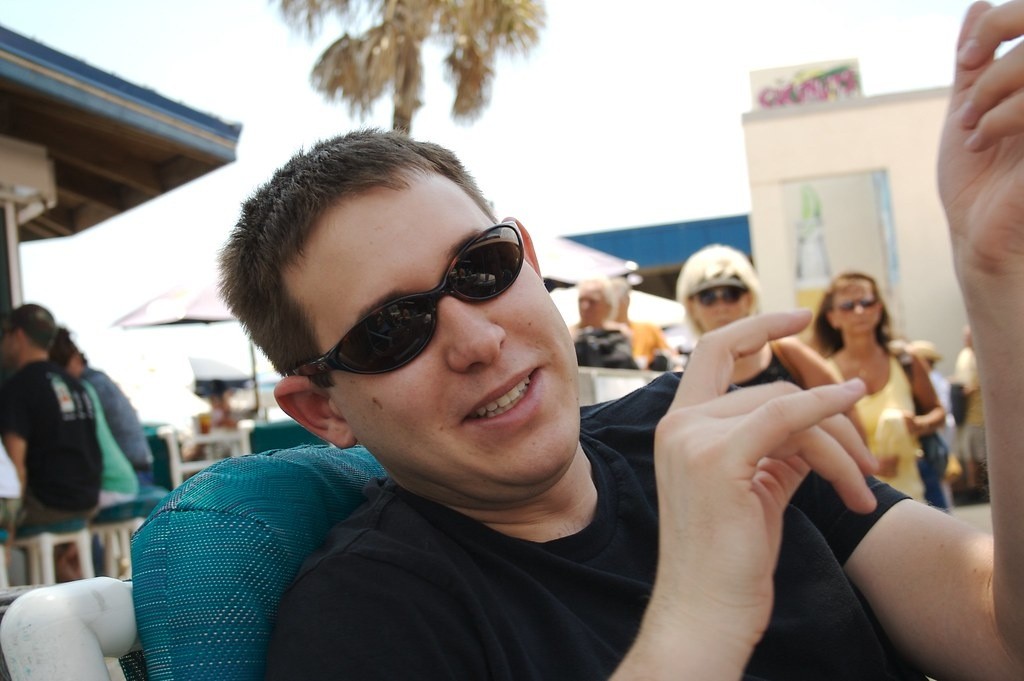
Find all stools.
<box><xmin>235</xmin><ymin>417</ymin><xmax>326</xmax><ymax>455</ymax></box>
<box><xmin>0</xmin><ymin>484</ymin><xmax>170</xmax><ymax>617</ymax></box>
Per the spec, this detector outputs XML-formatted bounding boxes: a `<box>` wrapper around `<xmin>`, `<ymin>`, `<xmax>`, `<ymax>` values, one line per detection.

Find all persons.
<box><xmin>1</xmin><ymin>303</ymin><xmax>153</xmax><ymax>543</ymax></box>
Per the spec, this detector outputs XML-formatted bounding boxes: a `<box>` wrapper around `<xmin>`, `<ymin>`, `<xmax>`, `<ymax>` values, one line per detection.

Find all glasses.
<box><xmin>836</xmin><ymin>297</ymin><xmax>881</xmax><ymax>311</ymax></box>
<box><xmin>286</xmin><ymin>221</ymin><xmax>524</xmax><ymax>378</ymax></box>
<box><xmin>694</xmin><ymin>286</ymin><xmax>747</xmax><ymax>306</ymax></box>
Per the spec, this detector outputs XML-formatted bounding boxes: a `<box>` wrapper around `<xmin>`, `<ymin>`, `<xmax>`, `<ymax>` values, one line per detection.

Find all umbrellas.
<box><xmin>505</xmin><ymin>230</ymin><xmax>688</xmax><ymax>330</ymax></box>
<box><xmin>109</xmin><ymin>281</ymin><xmax>263</xmax><ymax>416</ymax></box>
<box><xmin>179</xmin><ymin>0</ymin><xmax>1024</xmax><ymax>681</ymax></box>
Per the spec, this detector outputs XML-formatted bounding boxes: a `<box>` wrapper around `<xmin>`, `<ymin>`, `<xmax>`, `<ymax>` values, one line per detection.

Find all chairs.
<box><xmin>0</xmin><ymin>444</ymin><xmax>386</xmax><ymax>681</ymax></box>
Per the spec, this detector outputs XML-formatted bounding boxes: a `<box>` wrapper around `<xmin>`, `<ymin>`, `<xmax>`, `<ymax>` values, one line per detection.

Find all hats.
<box><xmin>677</xmin><ymin>244</ymin><xmax>759</xmax><ymax>310</ymax></box>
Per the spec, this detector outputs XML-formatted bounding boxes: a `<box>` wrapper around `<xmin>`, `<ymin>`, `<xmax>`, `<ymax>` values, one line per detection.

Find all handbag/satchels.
<box><xmin>900</xmin><ymin>351</ymin><xmax>948</xmax><ymax>478</ymax></box>
